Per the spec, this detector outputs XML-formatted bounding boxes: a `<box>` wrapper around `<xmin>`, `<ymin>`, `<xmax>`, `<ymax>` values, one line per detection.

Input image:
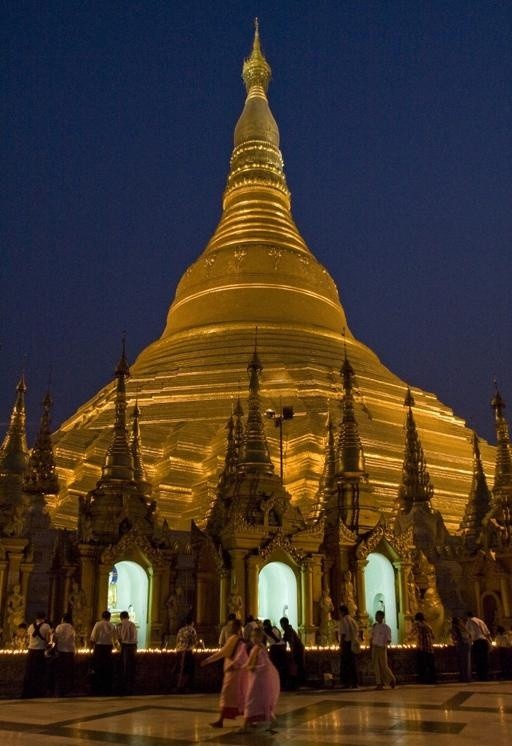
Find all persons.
<box><xmin>114</xmin><ymin>611</ymin><xmax>138</xmax><ymax>670</ymax></box>
<box><xmin>89</xmin><ymin>611</ymin><xmax>120</xmax><ymax>694</ymax></box>
<box><xmin>464</xmin><ymin>611</ymin><xmax>491</xmax><ymax>680</ymax></box>
<box><xmin>174</xmin><ymin>617</ymin><xmax>199</xmax><ymax>694</ymax></box>
<box><xmin>337</xmin><ymin>605</ymin><xmax>359</xmax><ymax>688</ymax></box>
<box><xmin>10</xmin><ymin>624</ymin><xmax>29</xmax><ymax>650</ymax></box>
<box><xmin>27</xmin><ymin>610</ymin><xmax>52</xmax><ymax>656</ymax></box>
<box><xmin>200</xmin><ymin>613</ymin><xmax>309</xmax><ymax>734</ymax></box>
<box><xmin>369</xmin><ymin>611</ymin><xmax>396</xmax><ymax>689</ymax></box>
<box><xmin>69</xmin><ymin>582</ymin><xmax>86</xmax><ymax>625</ymax></box>
<box><xmin>343</xmin><ymin>569</ymin><xmax>358</xmax><ymax>616</ymax></box>
<box><xmin>166</xmin><ymin>584</ymin><xmax>191</xmax><ymax>634</ymax></box>
<box><xmin>495</xmin><ymin>625</ymin><xmax>512</xmax><ymax>680</ymax></box>
<box><xmin>52</xmin><ymin>613</ymin><xmax>75</xmax><ymax>678</ymax></box>
<box><xmin>404</xmin><ymin>613</ymin><xmax>434</xmax><ymax>684</ymax></box>
<box><xmin>7</xmin><ymin>584</ymin><xmax>26</xmax><ymax>626</ymax></box>
<box><xmin>451</xmin><ymin>617</ymin><xmax>469</xmax><ymax>681</ymax></box>
<box><xmin>319</xmin><ymin>587</ymin><xmax>335</xmax><ymax>642</ymax></box>
<box><xmin>227</xmin><ymin>584</ymin><xmax>243</xmax><ymax>620</ymax></box>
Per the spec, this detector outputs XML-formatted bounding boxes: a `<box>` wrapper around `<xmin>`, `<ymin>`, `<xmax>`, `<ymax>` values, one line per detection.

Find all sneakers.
<box><xmin>339</xmin><ymin>678</ymin><xmax>396</xmax><ymax>690</ymax></box>
<box><xmin>208</xmin><ymin>721</ymin><xmax>223</xmax><ymax>728</ymax></box>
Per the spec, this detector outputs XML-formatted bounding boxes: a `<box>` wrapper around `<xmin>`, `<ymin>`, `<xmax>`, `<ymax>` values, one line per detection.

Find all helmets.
<box><xmin>234</xmin><ymin>719</ymin><xmax>280</xmax><ymax>735</ymax></box>
